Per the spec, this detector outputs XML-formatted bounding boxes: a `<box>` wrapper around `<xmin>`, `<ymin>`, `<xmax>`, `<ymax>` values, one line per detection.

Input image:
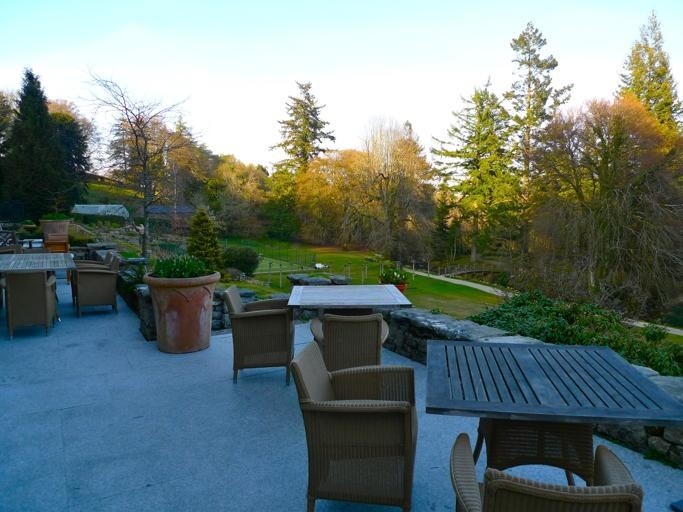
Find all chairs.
<box><xmin>447</xmin><ymin>428</ymin><xmax>647</xmax><ymax>511</ymax></box>
<box><xmin>285</xmin><ymin>338</ymin><xmax>418</xmax><ymax>510</ymax></box>
<box><xmin>222</xmin><ymin>282</ymin><xmax>293</xmax><ymax>386</ymax></box>
<box><xmin>308</xmin><ymin>312</ymin><xmax>390</xmax><ymax>370</ymax></box>
<box><xmin>0</xmin><ymin>217</ymin><xmax>122</xmax><ymax>340</ymax></box>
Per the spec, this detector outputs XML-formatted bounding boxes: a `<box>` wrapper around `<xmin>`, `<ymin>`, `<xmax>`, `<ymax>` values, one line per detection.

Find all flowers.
<box><xmin>378</xmin><ymin>267</ymin><xmax>408</xmax><ymax>286</ymax></box>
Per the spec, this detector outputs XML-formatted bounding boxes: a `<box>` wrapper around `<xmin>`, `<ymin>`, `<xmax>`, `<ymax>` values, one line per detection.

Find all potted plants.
<box><xmin>143</xmin><ymin>252</ymin><xmax>222</xmax><ymax>355</ymax></box>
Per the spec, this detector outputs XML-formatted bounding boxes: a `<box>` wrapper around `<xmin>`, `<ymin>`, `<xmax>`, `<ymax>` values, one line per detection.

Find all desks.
<box><xmin>423</xmin><ymin>337</ymin><xmax>683</xmax><ymax>486</ymax></box>
<box><xmin>285</xmin><ymin>282</ymin><xmax>413</xmax><ymax>319</ymax></box>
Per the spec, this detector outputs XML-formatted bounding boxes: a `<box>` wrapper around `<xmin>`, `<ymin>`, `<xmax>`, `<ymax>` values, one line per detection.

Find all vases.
<box><xmin>394</xmin><ymin>283</ymin><xmax>406</xmax><ymax>294</ymax></box>
